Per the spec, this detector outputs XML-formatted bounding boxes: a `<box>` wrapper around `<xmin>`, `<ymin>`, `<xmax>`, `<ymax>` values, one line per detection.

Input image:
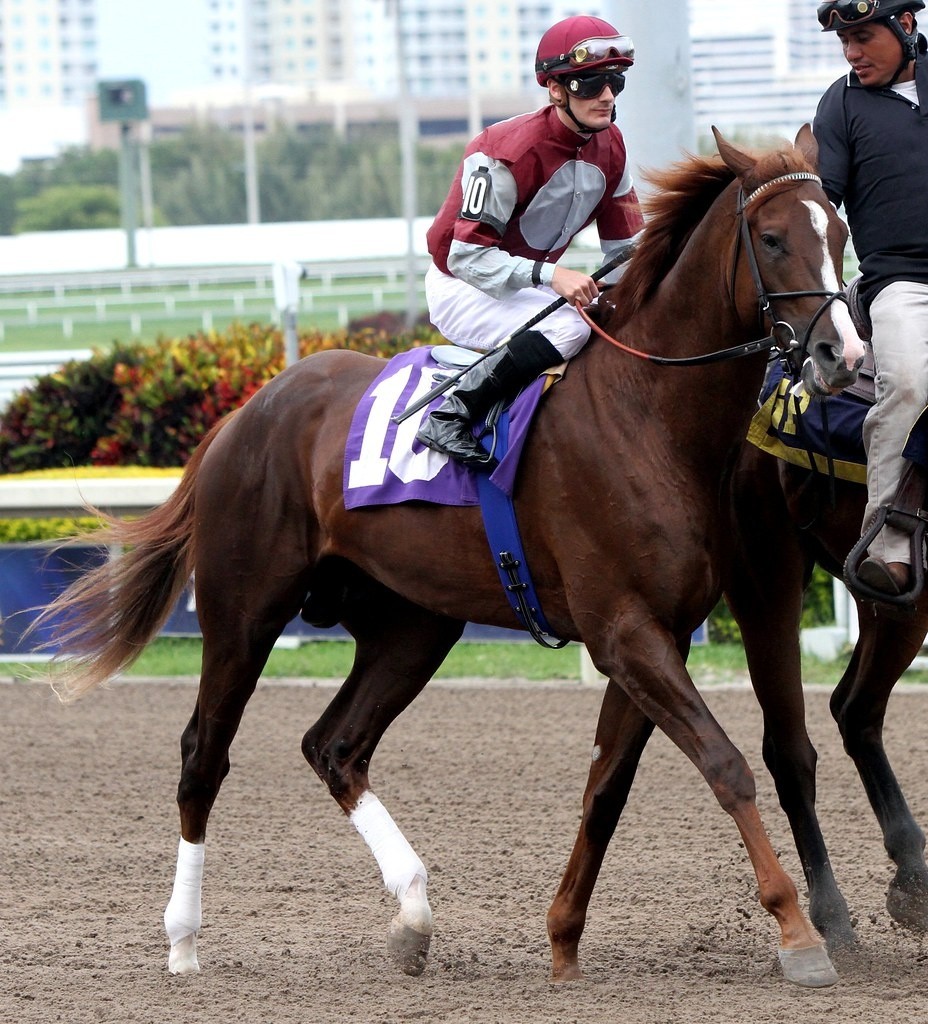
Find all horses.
<box><xmin>14</xmin><ymin>120</ymin><xmax>928</xmax><ymax>985</ymax></box>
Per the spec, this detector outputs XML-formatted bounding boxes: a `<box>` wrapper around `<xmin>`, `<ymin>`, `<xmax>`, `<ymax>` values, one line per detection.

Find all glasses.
<box><xmin>566</xmin><ymin>35</ymin><xmax>636</xmax><ymax>66</ymax></box>
<box><xmin>817</xmin><ymin>1</ymin><xmax>879</xmax><ymax>27</ymax></box>
<box><xmin>561</xmin><ymin>70</ymin><xmax>625</xmax><ymax>100</ymax></box>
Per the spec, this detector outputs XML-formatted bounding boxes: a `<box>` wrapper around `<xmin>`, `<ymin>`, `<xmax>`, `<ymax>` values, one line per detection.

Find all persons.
<box><xmin>426</xmin><ymin>18</ymin><xmax>646</xmax><ymax>464</ymax></box>
<box><xmin>813</xmin><ymin>0</ymin><xmax>928</xmax><ymax>595</ymax></box>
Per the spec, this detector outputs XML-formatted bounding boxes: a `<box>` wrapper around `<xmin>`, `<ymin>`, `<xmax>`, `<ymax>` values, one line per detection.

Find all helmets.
<box><xmin>817</xmin><ymin>0</ymin><xmax>926</xmax><ymax>87</ymax></box>
<box><xmin>536</xmin><ymin>15</ymin><xmax>636</xmax><ymax>134</ymax></box>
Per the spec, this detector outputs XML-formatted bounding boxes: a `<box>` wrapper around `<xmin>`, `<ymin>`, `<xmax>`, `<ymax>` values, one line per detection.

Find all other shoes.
<box><xmin>856</xmin><ymin>557</ymin><xmax>910</xmax><ymax>597</ymax></box>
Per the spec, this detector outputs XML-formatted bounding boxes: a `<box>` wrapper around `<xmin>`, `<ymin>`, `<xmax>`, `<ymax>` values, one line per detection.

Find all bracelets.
<box><xmin>532</xmin><ymin>260</ymin><xmax>547</xmax><ymax>287</ymax></box>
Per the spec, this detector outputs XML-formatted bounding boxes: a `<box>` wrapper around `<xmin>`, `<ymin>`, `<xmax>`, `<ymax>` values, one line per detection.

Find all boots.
<box><xmin>414</xmin><ymin>348</ymin><xmax>536</xmax><ymax>469</ymax></box>
